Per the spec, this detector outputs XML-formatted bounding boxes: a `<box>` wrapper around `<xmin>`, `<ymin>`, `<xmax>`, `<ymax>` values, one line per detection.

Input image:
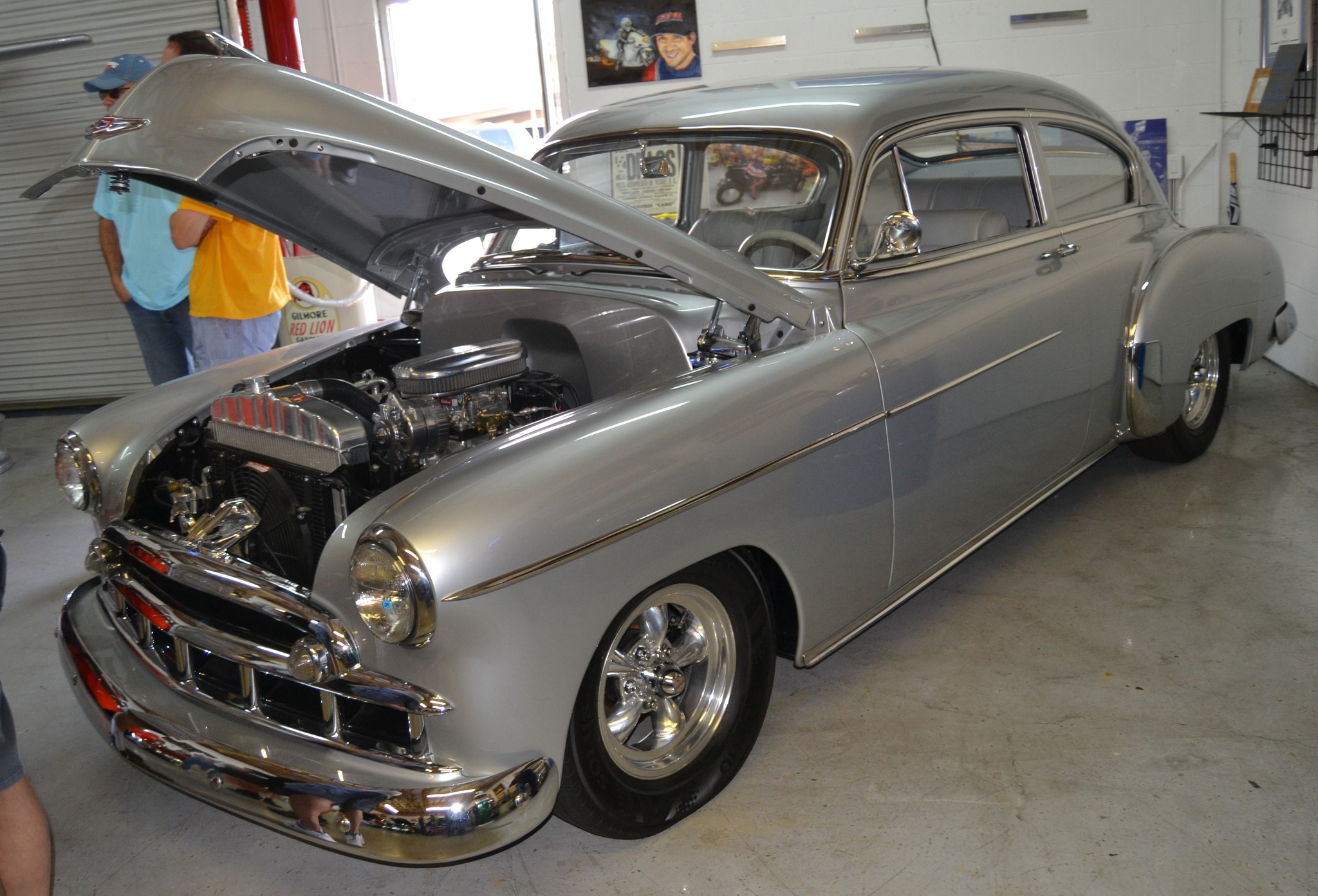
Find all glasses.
<box><xmin>98</xmin><ymin>88</ymin><xmax>133</xmax><ymax>101</ymax></box>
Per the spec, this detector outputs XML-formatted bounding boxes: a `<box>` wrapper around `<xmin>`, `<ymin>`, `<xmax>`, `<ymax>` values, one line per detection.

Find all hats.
<box><xmin>650</xmin><ymin>9</ymin><xmax>691</xmax><ymax>38</ymax></box>
<box><xmin>83</xmin><ymin>54</ymin><xmax>156</xmax><ymax>93</ymax></box>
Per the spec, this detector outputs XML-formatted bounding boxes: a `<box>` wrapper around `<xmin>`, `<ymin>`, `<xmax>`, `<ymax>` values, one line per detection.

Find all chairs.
<box><xmin>687</xmin><ymin>210</ymin><xmax>795</xmax><ymax>278</ymax></box>
<box><xmin>914</xmin><ymin>208</ymin><xmax>1010</xmax><ymax>257</ymax></box>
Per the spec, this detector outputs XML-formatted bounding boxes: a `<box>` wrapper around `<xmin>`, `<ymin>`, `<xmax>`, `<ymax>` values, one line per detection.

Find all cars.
<box><xmin>22</xmin><ymin>32</ymin><xmax>1297</xmax><ymax>867</ymax></box>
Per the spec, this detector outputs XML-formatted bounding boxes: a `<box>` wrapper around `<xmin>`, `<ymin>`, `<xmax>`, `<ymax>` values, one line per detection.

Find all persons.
<box><xmin>83</xmin><ymin>54</ymin><xmax>199</xmax><ymax>386</ymax></box>
<box><xmin>285</xmin><ymin>782</ymin><xmax>387</xmax><ymax>848</ymax></box>
<box><xmin>162</xmin><ymin>30</ymin><xmax>292</xmax><ymax>371</ymax></box>
<box><xmin>643</xmin><ymin>11</ymin><xmax>702</xmax><ymax>82</ymax></box>
<box><xmin>0</xmin><ymin>683</ymin><xmax>52</xmax><ymax>896</ymax></box>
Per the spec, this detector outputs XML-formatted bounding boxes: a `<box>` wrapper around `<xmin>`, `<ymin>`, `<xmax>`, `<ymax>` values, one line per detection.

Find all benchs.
<box><xmin>905</xmin><ymin>176</ymin><xmax>1034</xmax><ymax>232</ymax></box>
